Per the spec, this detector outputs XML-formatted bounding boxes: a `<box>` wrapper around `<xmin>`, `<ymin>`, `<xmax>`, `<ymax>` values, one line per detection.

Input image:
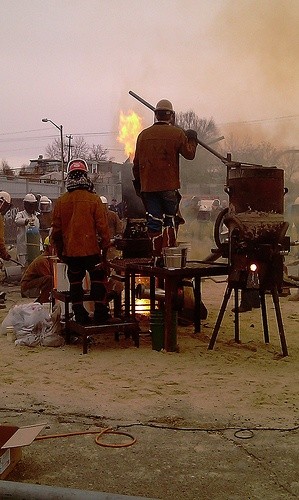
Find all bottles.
<box><xmin>6</xmin><ymin>322</ymin><xmax>15</xmax><ymax>343</ymax></box>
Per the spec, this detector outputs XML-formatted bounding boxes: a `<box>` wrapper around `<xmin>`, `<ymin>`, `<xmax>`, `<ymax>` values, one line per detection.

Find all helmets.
<box><xmin>23</xmin><ymin>194</ymin><xmax>38</xmax><ymax>202</ymax></box>
<box><xmin>65</xmin><ymin>159</ymin><xmax>89</xmax><ymax>173</ymax></box>
<box><xmin>212</xmin><ymin>199</ymin><xmax>221</xmax><ymax>205</ymax></box>
<box><xmin>43</xmin><ymin>236</ymin><xmax>50</xmax><ymax>246</ymax></box>
<box><xmin>100</xmin><ymin>196</ymin><xmax>108</xmax><ymax>203</ymax></box>
<box><xmin>0</xmin><ymin>192</ymin><xmax>11</xmax><ymax>203</ymax></box>
<box><xmin>155</xmin><ymin>99</ymin><xmax>174</xmax><ymax>113</ymax></box>
<box><xmin>40</xmin><ymin>196</ymin><xmax>51</xmax><ymax>202</ymax></box>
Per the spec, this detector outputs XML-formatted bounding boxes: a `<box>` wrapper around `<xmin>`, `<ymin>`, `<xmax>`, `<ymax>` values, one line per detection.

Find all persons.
<box><xmin>14</xmin><ymin>194</ymin><xmax>43</xmax><ymax>265</ymax></box>
<box><xmin>100</xmin><ymin>196</ymin><xmax>123</xmax><ymax>239</ymax></box>
<box><xmin>108</xmin><ymin>198</ymin><xmax>120</xmax><ymax>216</ymax></box>
<box><xmin>0</xmin><ymin>191</ymin><xmax>10</xmax><ymax>309</ymax></box>
<box><xmin>38</xmin><ymin>195</ymin><xmax>52</xmax><ymax>241</ymax></box>
<box><xmin>131</xmin><ymin>100</ymin><xmax>199</xmax><ymax>267</ymax></box>
<box><xmin>20</xmin><ymin>235</ymin><xmax>54</xmax><ymax>304</ymax></box>
<box><xmin>51</xmin><ymin>159</ymin><xmax>122</xmax><ymax>325</ymax></box>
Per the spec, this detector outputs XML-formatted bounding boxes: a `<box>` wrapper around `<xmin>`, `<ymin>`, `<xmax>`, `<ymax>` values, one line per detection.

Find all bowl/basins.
<box><xmin>162</xmin><ymin>247</ymin><xmax>188</xmax><ymax>270</ymax></box>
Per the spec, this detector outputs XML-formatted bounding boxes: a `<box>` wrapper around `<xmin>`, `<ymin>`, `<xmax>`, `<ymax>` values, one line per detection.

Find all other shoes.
<box><xmin>75</xmin><ymin>316</ymin><xmax>91</xmax><ymax>325</ymax></box>
<box><xmin>94</xmin><ymin>313</ymin><xmax>111</xmax><ymax>322</ymax></box>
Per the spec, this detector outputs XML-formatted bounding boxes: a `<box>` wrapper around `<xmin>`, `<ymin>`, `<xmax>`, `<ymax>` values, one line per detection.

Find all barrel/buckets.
<box><xmin>150</xmin><ymin>311</ymin><xmax>178</xmax><ymax>352</ymax></box>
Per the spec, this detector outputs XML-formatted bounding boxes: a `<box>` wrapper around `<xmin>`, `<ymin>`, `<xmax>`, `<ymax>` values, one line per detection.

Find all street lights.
<box><xmin>41</xmin><ymin>117</ymin><xmax>65</xmax><ymax>195</ymax></box>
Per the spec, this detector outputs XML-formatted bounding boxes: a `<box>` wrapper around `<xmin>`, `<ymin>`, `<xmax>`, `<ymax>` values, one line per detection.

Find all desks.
<box><xmin>125</xmin><ymin>259</ymin><xmax>240</xmax><ymax>352</ymax></box>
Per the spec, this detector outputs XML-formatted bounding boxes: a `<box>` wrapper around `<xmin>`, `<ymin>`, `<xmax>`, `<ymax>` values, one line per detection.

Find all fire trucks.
<box><xmin>182</xmin><ymin>194</ymin><xmax>219</xmax><ymax>221</ymax></box>
